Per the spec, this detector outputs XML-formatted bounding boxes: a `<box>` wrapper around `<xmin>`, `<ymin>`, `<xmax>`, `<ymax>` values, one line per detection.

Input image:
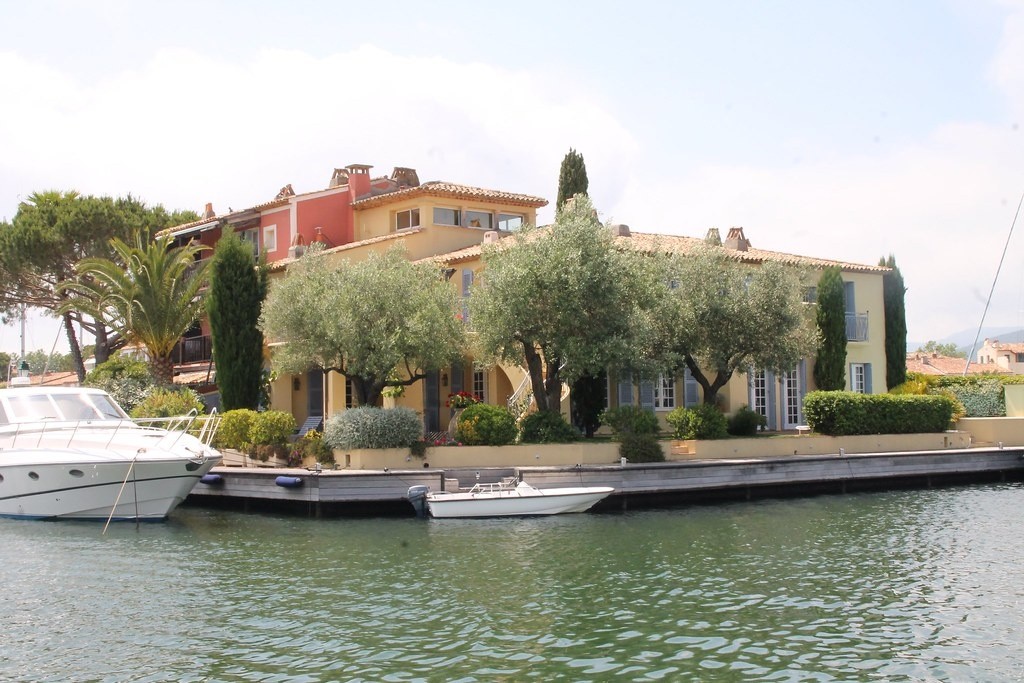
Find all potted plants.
<box><xmin>317</xmin><ymin>441</ymin><xmax>336</xmax><ymax>468</ymax></box>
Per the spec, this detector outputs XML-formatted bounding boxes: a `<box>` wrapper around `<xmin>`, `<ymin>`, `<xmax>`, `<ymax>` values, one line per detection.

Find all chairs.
<box><xmin>291</xmin><ymin>416</ymin><xmax>322</xmax><ymax>442</ymax></box>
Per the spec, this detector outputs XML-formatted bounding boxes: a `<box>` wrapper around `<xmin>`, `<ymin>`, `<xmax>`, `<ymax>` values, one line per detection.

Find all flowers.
<box><xmin>445</xmin><ymin>391</ymin><xmax>480</xmax><ymax>408</ymax></box>
<box><xmin>288</xmin><ymin>450</ymin><xmax>302</xmax><ymax>468</ymax></box>
<box><xmin>434</xmin><ymin>438</ymin><xmax>463</xmax><ymax>446</ymax></box>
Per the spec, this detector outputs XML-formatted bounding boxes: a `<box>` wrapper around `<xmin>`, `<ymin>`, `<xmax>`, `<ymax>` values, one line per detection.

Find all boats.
<box><xmin>406</xmin><ymin>468</ymin><xmax>615</xmax><ymax>520</ymax></box>
<box><xmin>0</xmin><ymin>316</ymin><xmax>225</xmax><ymax>535</ymax></box>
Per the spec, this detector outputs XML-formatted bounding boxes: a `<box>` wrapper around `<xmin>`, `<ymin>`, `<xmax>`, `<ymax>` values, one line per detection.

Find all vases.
<box><xmin>448</xmin><ymin>408</ymin><xmax>465</xmax><ymax>441</ymax></box>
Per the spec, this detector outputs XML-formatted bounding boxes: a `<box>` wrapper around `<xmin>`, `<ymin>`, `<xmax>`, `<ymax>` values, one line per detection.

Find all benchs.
<box><xmin>796</xmin><ymin>426</ymin><xmax>811</xmax><ymax>435</ymax></box>
